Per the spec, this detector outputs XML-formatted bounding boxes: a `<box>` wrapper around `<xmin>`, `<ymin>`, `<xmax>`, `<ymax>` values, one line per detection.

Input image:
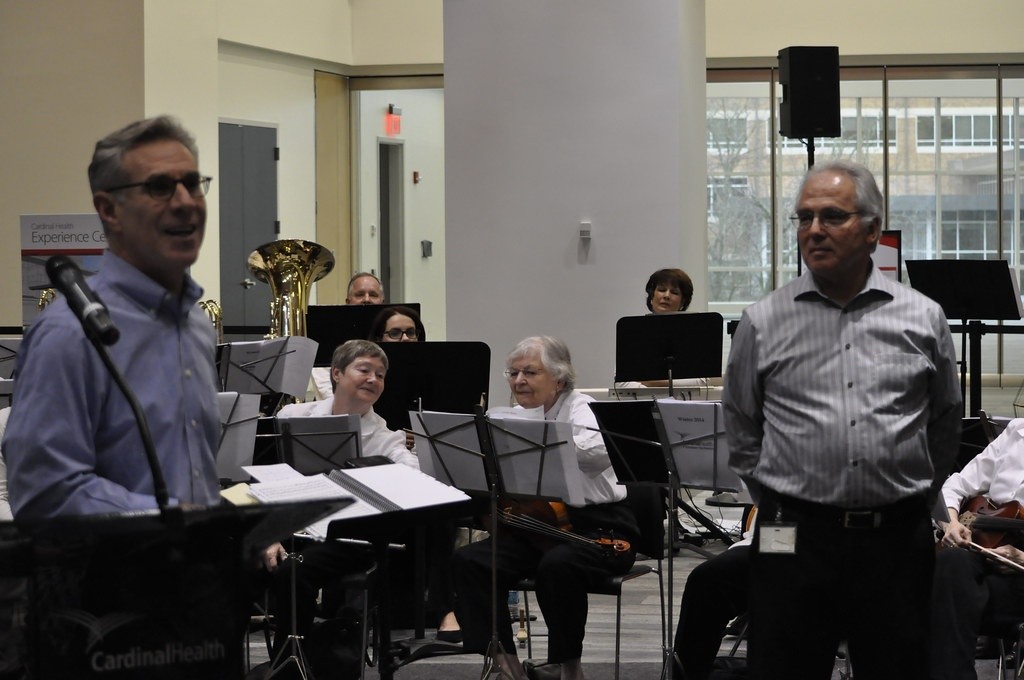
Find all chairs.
<box><xmin>509</xmin><ymin>483</ymin><xmax>668</xmax><ymax>680</ymax></box>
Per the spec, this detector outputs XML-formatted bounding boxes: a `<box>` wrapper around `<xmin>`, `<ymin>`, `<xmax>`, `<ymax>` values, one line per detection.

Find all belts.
<box><xmin>778</xmin><ymin>499</ymin><xmax>924</xmax><ymax>532</ymax></box>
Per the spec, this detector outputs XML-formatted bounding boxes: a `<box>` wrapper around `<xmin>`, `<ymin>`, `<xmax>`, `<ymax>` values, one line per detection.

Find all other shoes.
<box><xmin>508</xmin><ymin>604</ymin><xmax>537</xmax><ymax>621</ymax></box>
<box><xmin>975</xmin><ymin>637</ymin><xmax>1024</xmax><ymax>668</ymax></box>
<box><xmin>437</xmin><ymin>612</ymin><xmax>464</xmax><ymax>645</ymax></box>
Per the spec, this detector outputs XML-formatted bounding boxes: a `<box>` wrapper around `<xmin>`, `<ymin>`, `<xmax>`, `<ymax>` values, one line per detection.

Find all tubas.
<box><xmin>246</xmin><ymin>239</ymin><xmax>336</xmax><ymax>343</ymax></box>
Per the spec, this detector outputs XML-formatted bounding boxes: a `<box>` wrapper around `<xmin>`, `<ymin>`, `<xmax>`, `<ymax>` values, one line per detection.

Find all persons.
<box><xmin>0</xmin><ymin>116</ymin><xmax>221</xmax><ymax>520</ymax></box>
<box><xmin>720</xmin><ymin>161</ymin><xmax>963</xmax><ymax>680</ymax></box>
<box><xmin>0</xmin><ymin>267</ymin><xmax>1024</xmax><ymax>680</ymax></box>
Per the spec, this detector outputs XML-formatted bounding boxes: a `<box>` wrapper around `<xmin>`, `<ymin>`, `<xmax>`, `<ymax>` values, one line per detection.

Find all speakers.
<box><xmin>777</xmin><ymin>46</ymin><xmax>841</xmax><ymax>138</ymax></box>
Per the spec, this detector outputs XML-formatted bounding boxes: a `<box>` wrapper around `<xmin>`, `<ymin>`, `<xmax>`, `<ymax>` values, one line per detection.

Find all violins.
<box><xmin>479</xmin><ymin>496</ymin><xmax>631</xmax><ymax>563</ymax></box>
<box><xmin>935</xmin><ymin>496</ymin><xmax>1024</xmax><ymax>556</ymax></box>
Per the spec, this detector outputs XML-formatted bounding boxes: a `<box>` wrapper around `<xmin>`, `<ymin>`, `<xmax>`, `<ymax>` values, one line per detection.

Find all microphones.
<box><xmin>46</xmin><ymin>256</ymin><xmax>119</xmax><ymax>346</ymax></box>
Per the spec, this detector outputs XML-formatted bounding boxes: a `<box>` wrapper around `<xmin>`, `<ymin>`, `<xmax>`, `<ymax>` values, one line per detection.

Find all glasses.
<box><xmin>787</xmin><ymin>206</ymin><xmax>860</xmax><ymax>231</ymax></box>
<box><xmin>504</xmin><ymin>367</ymin><xmax>543</xmax><ymax>379</ymax></box>
<box><xmin>108</xmin><ymin>170</ymin><xmax>213</xmax><ymax>203</ymax></box>
<box><xmin>383</xmin><ymin>328</ymin><xmax>420</xmax><ymax>339</ymax></box>
